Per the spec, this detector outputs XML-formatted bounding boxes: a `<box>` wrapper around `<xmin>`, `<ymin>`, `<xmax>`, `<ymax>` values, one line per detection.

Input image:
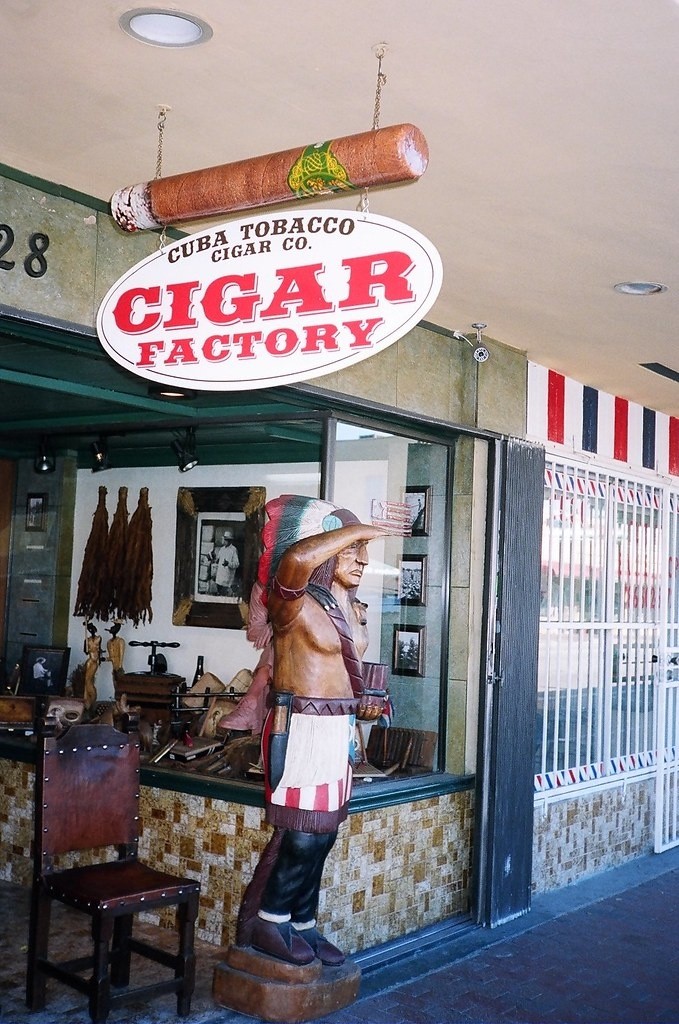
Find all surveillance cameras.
<box><xmin>472</xmin><ymin>342</ymin><xmax>490</xmax><ymax>362</ymax></box>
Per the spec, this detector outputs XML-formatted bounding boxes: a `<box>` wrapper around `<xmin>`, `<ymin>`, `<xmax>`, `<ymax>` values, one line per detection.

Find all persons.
<box><xmin>253</xmin><ymin>507</ymin><xmax>389</xmax><ymax>968</ymax></box>
<box><xmin>82</xmin><ymin>623</ymin><xmax>104</xmax><ymax>704</ymax></box>
<box><xmin>100</xmin><ymin>624</ymin><xmax>125</xmax><ymax>687</ymax></box>
<box><xmin>32</xmin><ymin>657</ymin><xmax>48</xmax><ymax>693</ymax></box>
<box><xmin>215</xmin><ymin>575</ymin><xmax>274</xmax><ymax>733</ymax></box>
<box><xmin>215</xmin><ymin>531</ymin><xmax>238</xmax><ymax>596</ymax></box>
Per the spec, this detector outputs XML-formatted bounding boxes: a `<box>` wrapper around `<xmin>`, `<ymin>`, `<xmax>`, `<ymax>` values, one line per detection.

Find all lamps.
<box><xmin>34</xmin><ymin>445</ymin><xmax>55</xmax><ymax>474</ymax></box>
<box><xmin>88</xmin><ymin>443</ymin><xmax>112</xmax><ymax>472</ymax></box>
<box><xmin>169</xmin><ymin>440</ymin><xmax>199</xmax><ymax>471</ymax></box>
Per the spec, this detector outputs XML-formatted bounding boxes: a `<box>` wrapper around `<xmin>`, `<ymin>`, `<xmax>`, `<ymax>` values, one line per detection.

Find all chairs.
<box><xmin>26</xmin><ymin>725</ymin><xmax>201</xmax><ymax>1024</ymax></box>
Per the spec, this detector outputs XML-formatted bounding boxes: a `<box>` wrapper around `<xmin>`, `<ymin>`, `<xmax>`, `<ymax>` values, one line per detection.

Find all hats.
<box><xmin>37</xmin><ymin>658</ymin><xmax>46</xmax><ymax>663</ymax></box>
<box><xmin>223</xmin><ymin>532</ymin><xmax>234</xmax><ymax>539</ymax></box>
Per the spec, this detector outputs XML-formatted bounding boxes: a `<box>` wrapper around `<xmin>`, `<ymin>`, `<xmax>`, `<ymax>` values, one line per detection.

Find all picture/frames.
<box><xmin>25</xmin><ymin>493</ymin><xmax>49</xmax><ymax>533</ymax></box>
<box><xmin>173</xmin><ymin>487</ymin><xmax>266</xmax><ymax>630</ymax></box>
<box><xmin>395</xmin><ymin>554</ymin><xmax>427</xmax><ymax>607</ymax></box>
<box><xmin>17</xmin><ymin>645</ymin><xmax>71</xmax><ymax>695</ymax></box>
<box><xmin>391</xmin><ymin>624</ymin><xmax>427</xmax><ymax>678</ymax></box>
<box><xmin>399</xmin><ymin>486</ymin><xmax>432</xmax><ymax>537</ymax></box>
